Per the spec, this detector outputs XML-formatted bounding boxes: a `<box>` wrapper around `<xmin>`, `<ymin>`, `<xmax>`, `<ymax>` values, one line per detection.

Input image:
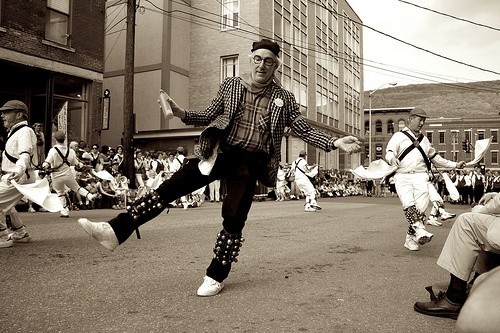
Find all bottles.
<box><xmin>159</xmin><ymin>89</ymin><xmax>173</xmax><ymax>120</ymax></box>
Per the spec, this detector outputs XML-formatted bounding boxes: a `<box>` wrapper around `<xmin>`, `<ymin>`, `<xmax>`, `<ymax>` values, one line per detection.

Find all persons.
<box><xmin>267</xmin><ymin>160</ymin><xmax>399</xmax><ymax>202</ymax></box>
<box><xmin>41</xmin><ymin>130</ymin><xmax>101</xmax><ymax>217</ymax></box>
<box><xmin>430</xmin><ymin>161</ymin><xmax>500</xmax><ymax>206</ymax></box>
<box><xmin>0</xmin><ymin>101</ymin><xmax>39</xmax><ymax>247</ymax></box>
<box><xmin>292</xmin><ymin>150</ymin><xmax>323</xmax><ymax>212</ymax></box>
<box><xmin>78</xmin><ymin>40</ymin><xmax>363</xmax><ymax>297</ymax></box>
<box><xmin>30</xmin><ymin>123</ymin><xmax>46</xmax><ymax>165</ymax></box>
<box><xmin>426</xmin><ymin>172</ymin><xmax>457</xmax><ymax>226</ymax></box>
<box><xmin>26</xmin><ymin>200</ymin><xmax>48</xmax><ymax>213</ymax></box>
<box><xmin>67</xmin><ymin>139</ymin><xmax>226</xmax><ymax>210</ymax></box>
<box><xmin>384</xmin><ymin>108</ymin><xmax>467</xmax><ymax>250</ymax></box>
<box><xmin>413</xmin><ymin>191</ymin><xmax>500</xmax><ymax>333</ymax></box>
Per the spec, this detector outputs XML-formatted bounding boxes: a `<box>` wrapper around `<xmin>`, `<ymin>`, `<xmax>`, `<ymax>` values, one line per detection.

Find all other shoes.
<box><xmin>29</xmin><ymin>209</ymin><xmax>35</xmax><ymax>213</ymax></box>
<box><xmin>60</xmin><ymin>215</ymin><xmax>68</xmax><ymax>218</ymax></box>
<box><xmin>90</xmin><ymin>194</ymin><xmax>99</xmax><ymax>200</ymax></box>
<box><xmin>39</xmin><ymin>208</ymin><xmax>46</xmax><ymax>212</ymax></box>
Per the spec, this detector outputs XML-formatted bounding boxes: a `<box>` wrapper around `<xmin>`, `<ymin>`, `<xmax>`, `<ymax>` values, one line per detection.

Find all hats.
<box><xmin>409</xmin><ymin>108</ymin><xmax>429</xmax><ymax>118</ymax></box>
<box><xmin>300</xmin><ymin>150</ymin><xmax>308</xmax><ymax>155</ymax></box>
<box><xmin>54</xmin><ymin>131</ymin><xmax>65</xmax><ymax>139</ymax></box>
<box><xmin>0</xmin><ymin>100</ymin><xmax>28</xmax><ymax>113</ymax></box>
<box><xmin>251</xmin><ymin>39</ymin><xmax>281</xmax><ymax>58</ymax></box>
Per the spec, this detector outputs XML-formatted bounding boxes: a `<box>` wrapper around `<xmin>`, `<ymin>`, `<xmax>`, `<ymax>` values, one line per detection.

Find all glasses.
<box><xmin>252</xmin><ymin>55</ymin><xmax>278</xmax><ymax>66</ymax></box>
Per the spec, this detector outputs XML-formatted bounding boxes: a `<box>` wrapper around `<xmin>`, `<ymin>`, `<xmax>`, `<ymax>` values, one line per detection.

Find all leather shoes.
<box><xmin>467</xmin><ymin>283</ymin><xmax>472</xmax><ymax>294</ymax></box>
<box><xmin>414</xmin><ymin>295</ymin><xmax>462</xmax><ymax>320</ymax></box>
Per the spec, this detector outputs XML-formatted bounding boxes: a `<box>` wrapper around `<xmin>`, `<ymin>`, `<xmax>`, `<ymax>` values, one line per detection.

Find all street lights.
<box><xmin>369</xmin><ymin>82</ymin><xmax>397</xmax><ymax>164</ymax></box>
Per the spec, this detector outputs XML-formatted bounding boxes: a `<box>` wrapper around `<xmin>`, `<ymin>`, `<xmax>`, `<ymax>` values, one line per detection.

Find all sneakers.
<box><xmin>404</xmin><ymin>236</ymin><xmax>420</xmax><ymax>251</ymax></box>
<box><xmin>426</xmin><ymin>220</ymin><xmax>442</xmax><ymax>226</ymax></box>
<box><xmin>0</xmin><ymin>236</ymin><xmax>13</xmax><ymax>248</ymax></box>
<box><xmin>78</xmin><ymin>218</ymin><xmax>119</xmax><ymax>252</ymax></box>
<box><xmin>196</xmin><ymin>275</ymin><xmax>225</xmax><ymax>296</ymax></box>
<box><xmin>417</xmin><ymin>230</ymin><xmax>434</xmax><ymax>245</ymax></box>
<box><xmin>441</xmin><ymin>213</ymin><xmax>456</xmax><ymax>221</ymax></box>
<box><xmin>6</xmin><ymin>232</ymin><xmax>30</xmax><ymax>243</ymax></box>
<box><xmin>305</xmin><ymin>206</ymin><xmax>316</xmax><ymax>212</ymax></box>
<box><xmin>310</xmin><ymin>204</ymin><xmax>322</xmax><ymax>210</ymax></box>
